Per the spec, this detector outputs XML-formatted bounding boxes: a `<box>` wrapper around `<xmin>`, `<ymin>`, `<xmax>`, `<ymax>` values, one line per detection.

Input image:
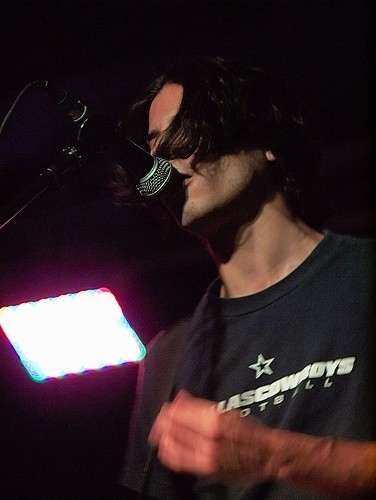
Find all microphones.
<box><xmin>44</xmin><ymin>81</ymin><xmax>174</xmax><ymax>197</ymax></box>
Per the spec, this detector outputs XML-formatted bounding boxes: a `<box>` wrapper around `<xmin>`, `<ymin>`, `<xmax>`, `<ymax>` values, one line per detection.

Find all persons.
<box><xmin>111</xmin><ymin>58</ymin><xmax>376</xmax><ymax>500</ymax></box>
